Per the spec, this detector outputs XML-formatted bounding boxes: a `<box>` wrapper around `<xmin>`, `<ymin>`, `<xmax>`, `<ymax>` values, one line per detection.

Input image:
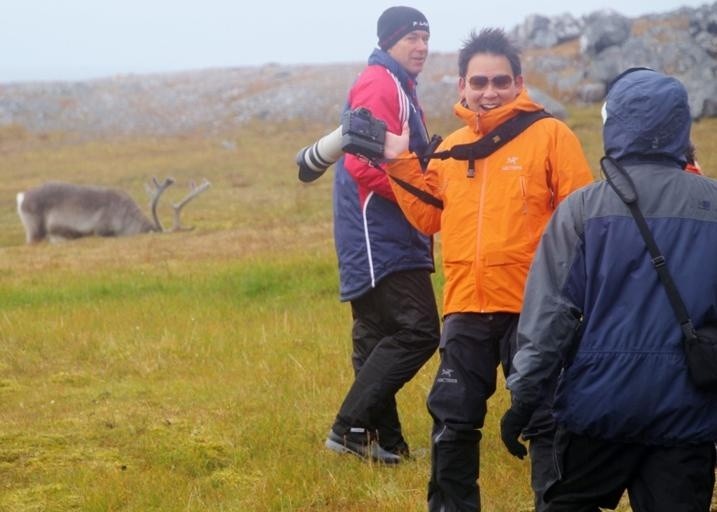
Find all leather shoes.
<box><xmin>326</xmin><ymin>420</ymin><xmax>416</xmax><ymax>467</ymax></box>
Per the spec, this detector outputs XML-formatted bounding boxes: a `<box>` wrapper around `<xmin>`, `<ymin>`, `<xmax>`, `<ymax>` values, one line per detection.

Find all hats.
<box><xmin>377</xmin><ymin>7</ymin><xmax>430</xmax><ymax>51</ymax></box>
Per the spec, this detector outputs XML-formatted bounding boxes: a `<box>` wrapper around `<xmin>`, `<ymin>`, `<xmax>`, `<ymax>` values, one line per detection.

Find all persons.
<box><xmin>323</xmin><ymin>4</ymin><xmax>443</xmax><ymax>470</ymax></box>
<box><xmin>497</xmin><ymin>64</ymin><xmax>715</xmax><ymax>510</ymax></box>
<box><xmin>356</xmin><ymin>28</ymin><xmax>594</xmax><ymax>512</ymax></box>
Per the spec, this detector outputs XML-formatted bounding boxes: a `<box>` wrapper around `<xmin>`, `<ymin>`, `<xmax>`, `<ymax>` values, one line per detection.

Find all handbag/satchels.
<box><xmin>683</xmin><ymin>324</ymin><xmax>717</xmax><ymax>389</ymax></box>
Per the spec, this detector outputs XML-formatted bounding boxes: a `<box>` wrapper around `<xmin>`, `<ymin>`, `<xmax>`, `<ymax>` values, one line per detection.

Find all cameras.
<box><xmin>296</xmin><ymin>107</ymin><xmax>386</xmax><ymax>183</ymax></box>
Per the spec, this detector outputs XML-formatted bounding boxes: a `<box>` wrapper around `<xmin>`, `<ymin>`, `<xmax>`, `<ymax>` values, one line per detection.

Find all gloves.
<box><xmin>500</xmin><ymin>408</ymin><xmax>531</xmax><ymax>460</ymax></box>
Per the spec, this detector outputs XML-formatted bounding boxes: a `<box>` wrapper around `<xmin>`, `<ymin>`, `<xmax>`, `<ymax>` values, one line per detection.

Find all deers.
<box><xmin>16</xmin><ymin>177</ymin><xmax>212</xmax><ymax>244</ymax></box>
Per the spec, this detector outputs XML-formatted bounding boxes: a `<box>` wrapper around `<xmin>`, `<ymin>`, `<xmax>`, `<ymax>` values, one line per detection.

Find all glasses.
<box><xmin>464</xmin><ymin>75</ymin><xmax>513</xmax><ymax>91</ymax></box>
